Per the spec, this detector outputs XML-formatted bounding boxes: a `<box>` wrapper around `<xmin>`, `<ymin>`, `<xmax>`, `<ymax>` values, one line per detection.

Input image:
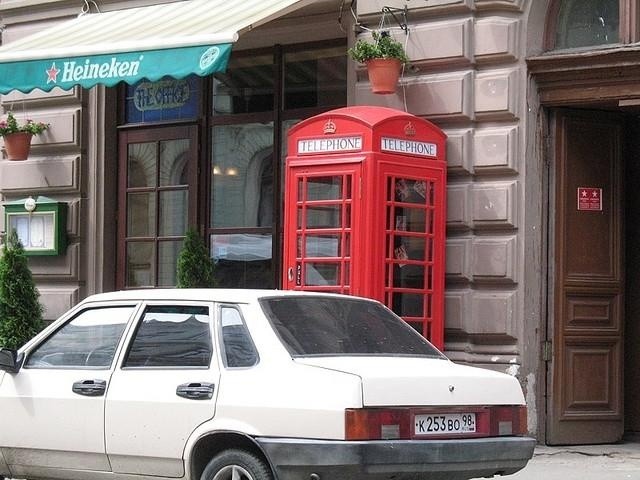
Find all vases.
<box><xmin>3</xmin><ymin>131</ymin><xmax>33</xmax><ymax>161</ymax></box>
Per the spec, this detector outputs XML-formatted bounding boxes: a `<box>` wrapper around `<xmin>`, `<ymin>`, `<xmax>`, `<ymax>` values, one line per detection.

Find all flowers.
<box><xmin>0</xmin><ymin>111</ymin><xmax>51</xmax><ymax>137</ymax></box>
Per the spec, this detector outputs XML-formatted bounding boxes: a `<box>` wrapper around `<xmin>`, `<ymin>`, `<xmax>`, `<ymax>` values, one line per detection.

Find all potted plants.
<box><xmin>346</xmin><ymin>30</ymin><xmax>419</xmax><ymax>94</ymax></box>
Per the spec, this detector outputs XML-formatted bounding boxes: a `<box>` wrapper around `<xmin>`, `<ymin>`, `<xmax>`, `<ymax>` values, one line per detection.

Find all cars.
<box><xmin>0</xmin><ymin>289</ymin><xmax>536</xmax><ymax>480</ymax></box>
